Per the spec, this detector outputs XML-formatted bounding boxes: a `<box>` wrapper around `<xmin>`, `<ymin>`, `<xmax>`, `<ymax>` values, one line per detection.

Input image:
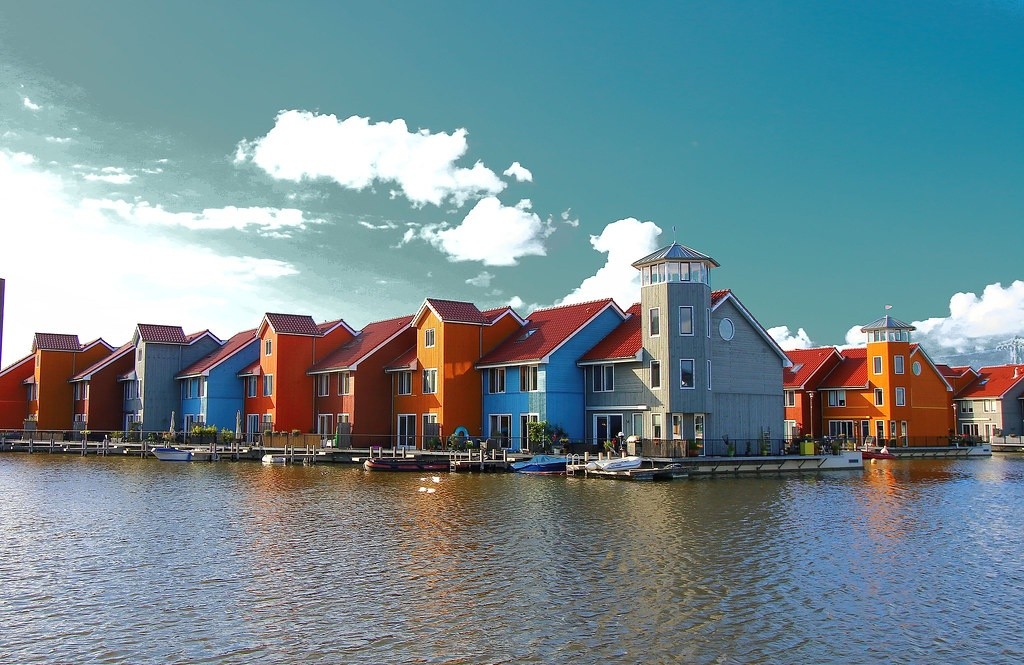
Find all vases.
<box><xmin>562</xmin><ymin>447</ymin><xmax>568</xmax><ymax>455</ymax></box>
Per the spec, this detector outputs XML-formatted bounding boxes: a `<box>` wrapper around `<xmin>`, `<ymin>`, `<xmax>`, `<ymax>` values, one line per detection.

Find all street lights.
<box><xmin>809</xmin><ymin>391</ymin><xmax>814</xmax><ymax>441</ymax></box>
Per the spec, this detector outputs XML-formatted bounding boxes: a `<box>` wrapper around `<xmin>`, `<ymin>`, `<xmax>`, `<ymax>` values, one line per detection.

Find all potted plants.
<box><xmin>552</xmin><ymin>446</ymin><xmax>561</xmax><ymax>455</ymax></box>
<box><xmin>603</xmin><ymin>441</ymin><xmax>612</xmax><ymax>456</ymax></box>
<box><xmin>758</xmin><ymin>432</ymin><xmax>768</xmax><ymax>456</ymax></box>
<box><xmin>109</xmin><ymin>432</ymin><xmax>128</xmax><ymax>443</ymax></box>
<box><xmin>526</xmin><ymin>420</ymin><xmax>553</xmax><ymax>454</ymax></box>
<box><xmin>279</xmin><ymin>431</ymin><xmax>288</xmax><ymax>437</ymax></box>
<box><xmin>831</xmin><ymin>441</ymin><xmax>840</xmax><ymax>455</ymax></box>
<box><xmin>291</xmin><ymin>429</ymin><xmax>302</xmax><ymax>437</ymax></box>
<box><xmin>727</xmin><ymin>442</ymin><xmax>735</xmax><ymax>457</ymax></box>
<box><xmin>780</xmin><ymin>448</ymin><xmax>784</xmax><ymax>455</ymax></box>
<box><xmin>745</xmin><ymin>442</ymin><xmax>754</xmax><ymax>457</ymax></box>
<box><xmin>262</xmin><ymin>430</ymin><xmax>279</xmax><ymax>438</ymax></box>
<box><xmin>429</xmin><ymin>434</ymin><xmax>442</xmax><ymax>451</ymax></box>
<box><xmin>686</xmin><ymin>438</ymin><xmax>703</xmax><ymax>457</ymax></box>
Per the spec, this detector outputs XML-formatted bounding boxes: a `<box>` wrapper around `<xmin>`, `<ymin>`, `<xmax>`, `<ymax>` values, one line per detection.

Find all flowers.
<box><xmin>559</xmin><ymin>438</ymin><xmax>569</xmax><ymax>448</ymax></box>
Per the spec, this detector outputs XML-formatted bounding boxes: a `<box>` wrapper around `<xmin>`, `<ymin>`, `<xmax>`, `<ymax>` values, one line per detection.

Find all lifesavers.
<box><xmin>454</xmin><ymin>426</ymin><xmax>468</xmax><ymax>436</ymax></box>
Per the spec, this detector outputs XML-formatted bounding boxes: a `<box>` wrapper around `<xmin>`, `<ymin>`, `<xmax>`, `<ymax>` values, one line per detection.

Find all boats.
<box><xmin>509</xmin><ymin>455</ymin><xmax>578</xmax><ymax>476</ymax></box>
<box><xmin>363</xmin><ymin>457</ymin><xmax>469</xmax><ymax>471</ymax></box>
<box><xmin>150</xmin><ymin>447</ymin><xmax>221</xmax><ymax>461</ymax></box>
<box><xmin>585</xmin><ymin>456</ymin><xmax>642</xmax><ymax>472</ymax></box>
<box><xmin>262</xmin><ymin>454</ymin><xmax>285</xmax><ymax>462</ymax></box>
